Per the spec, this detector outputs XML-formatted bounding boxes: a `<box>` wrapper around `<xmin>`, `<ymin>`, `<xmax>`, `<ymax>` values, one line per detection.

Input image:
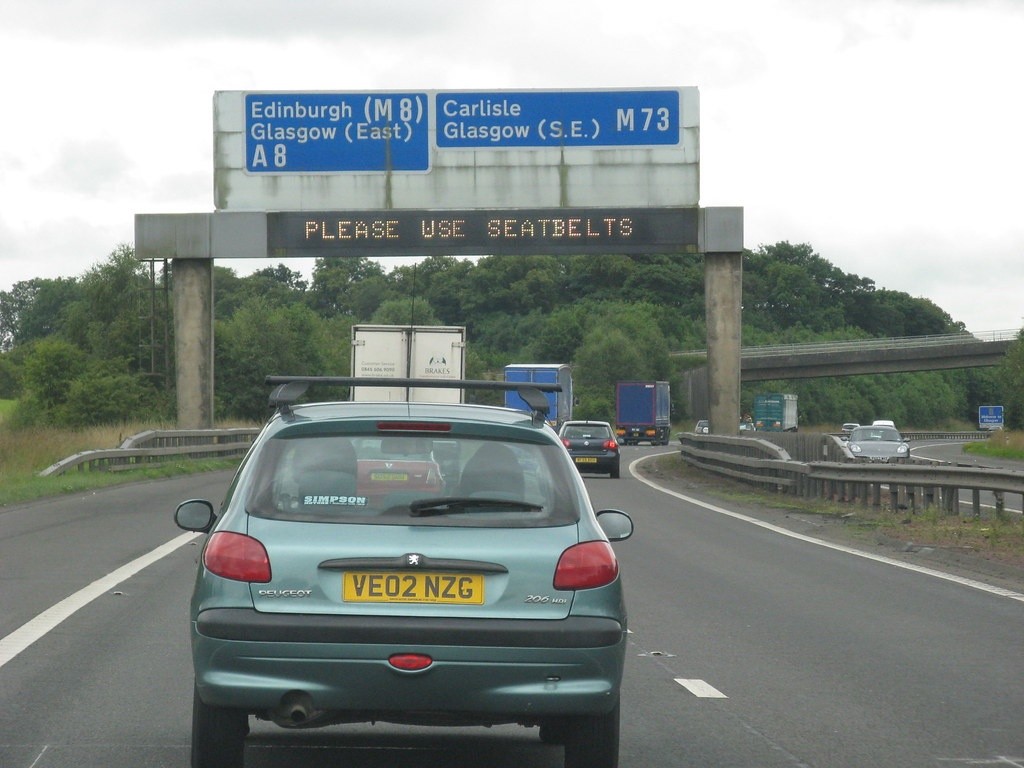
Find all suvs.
<box><xmin>740</xmin><ymin>422</ymin><xmax>758</xmax><ymax>432</ymax></box>
<box><xmin>694</xmin><ymin>418</ymin><xmax>709</xmax><ymax>434</ymax></box>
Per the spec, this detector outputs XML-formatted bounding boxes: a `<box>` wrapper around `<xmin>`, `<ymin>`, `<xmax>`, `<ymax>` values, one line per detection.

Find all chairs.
<box><xmin>297</xmin><ymin>472</ymin><xmax>367</xmax><ymax>518</ymax></box>
<box><xmin>456</xmin><ymin>462</ymin><xmax>526</xmax><ymax>509</ymax></box>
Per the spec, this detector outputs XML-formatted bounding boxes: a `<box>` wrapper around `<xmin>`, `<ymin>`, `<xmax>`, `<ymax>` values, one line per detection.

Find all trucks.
<box><xmin>502</xmin><ymin>363</ymin><xmax>581</xmax><ymax>436</ymax></box>
<box><xmin>615</xmin><ymin>380</ymin><xmax>676</xmax><ymax>446</ymax></box>
<box><xmin>753</xmin><ymin>393</ymin><xmax>802</xmax><ymax>433</ymax></box>
<box><xmin>347</xmin><ymin>323</ymin><xmax>467</xmax><ymax>458</ymax></box>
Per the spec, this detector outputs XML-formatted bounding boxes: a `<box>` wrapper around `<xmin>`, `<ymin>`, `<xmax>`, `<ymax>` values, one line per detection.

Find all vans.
<box><xmin>870</xmin><ymin>420</ymin><xmax>897</xmax><ymax>438</ymax></box>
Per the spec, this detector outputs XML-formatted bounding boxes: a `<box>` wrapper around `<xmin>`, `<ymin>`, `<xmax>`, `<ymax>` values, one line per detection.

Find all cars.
<box><xmin>842</xmin><ymin>421</ymin><xmax>860</xmax><ymax>432</ymax></box>
<box><xmin>559</xmin><ymin>420</ymin><xmax>625</xmax><ymax>479</ymax></box>
<box><xmin>174</xmin><ymin>375</ymin><xmax>636</xmax><ymax>768</ymax></box>
<box><xmin>840</xmin><ymin>425</ymin><xmax>911</xmax><ymax>463</ymax></box>
<box><xmin>350</xmin><ymin>436</ymin><xmax>446</xmax><ymax>499</ymax></box>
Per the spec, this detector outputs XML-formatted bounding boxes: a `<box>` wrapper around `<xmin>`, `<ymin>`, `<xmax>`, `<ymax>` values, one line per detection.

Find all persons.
<box><xmin>298</xmin><ymin>456</ymin><xmax>354</xmax><ymax>515</ymax></box>
<box><xmin>450</xmin><ymin>444</ymin><xmax>526</xmax><ymax>510</ymax></box>
<box><xmin>747</xmin><ymin>414</ymin><xmax>752</xmax><ymax>422</ymax></box>
